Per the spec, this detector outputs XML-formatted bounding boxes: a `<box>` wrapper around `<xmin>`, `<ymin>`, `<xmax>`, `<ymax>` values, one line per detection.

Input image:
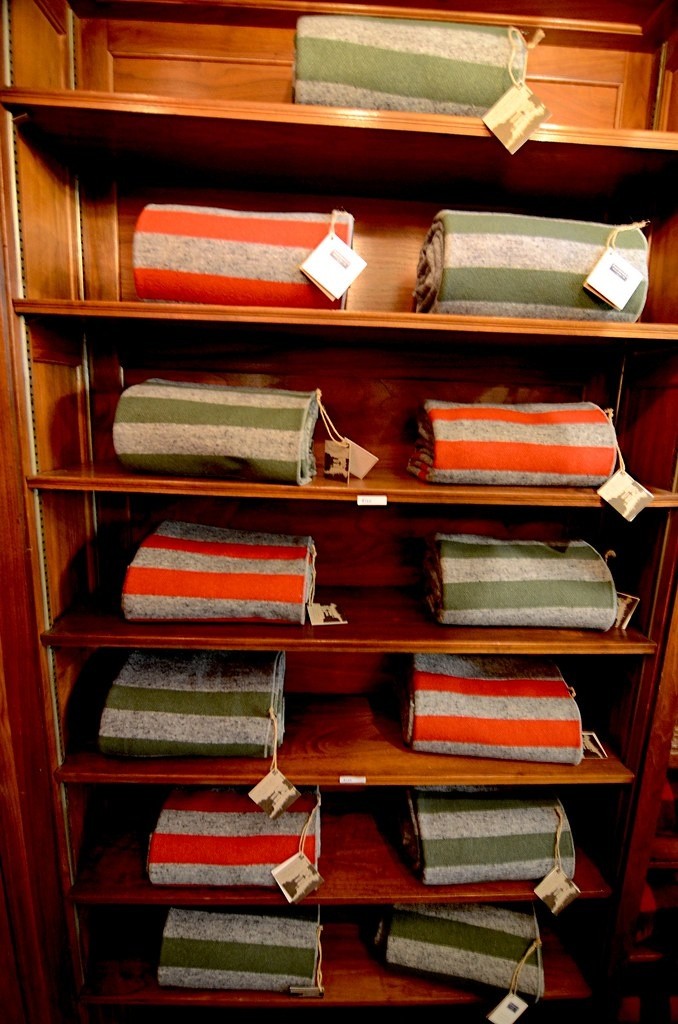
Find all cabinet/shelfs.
<box><xmin>0</xmin><ymin>0</ymin><xmax>677</xmax><ymax>1024</ymax></box>
